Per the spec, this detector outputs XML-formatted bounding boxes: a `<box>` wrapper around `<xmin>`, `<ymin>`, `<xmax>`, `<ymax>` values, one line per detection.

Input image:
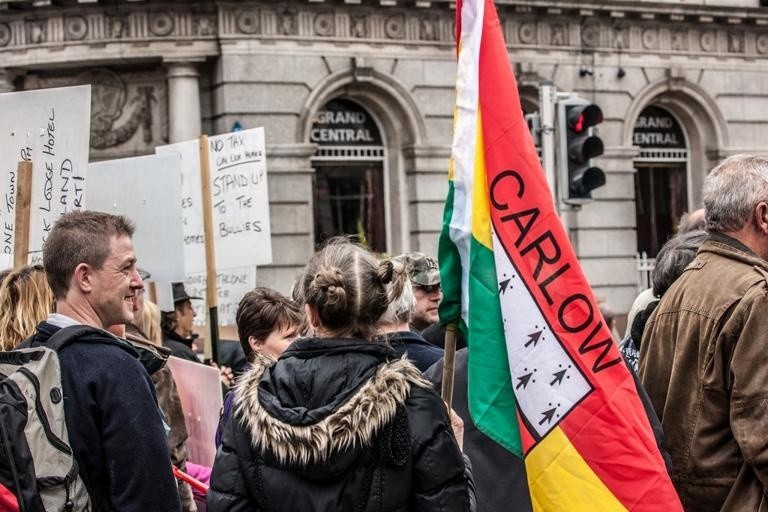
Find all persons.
<box><xmin>625</xmin><ymin>210</ymin><xmax>708</xmax><ymax>342</ymax></box>
<box><xmin>208</xmin><ymin>237</ymin><xmax>530</xmax><ymax>511</ymax></box>
<box><xmin>631</xmin><ymin>230</ymin><xmax>712</xmax><ymax>354</ymax></box>
<box><xmin>638</xmin><ymin>154</ymin><xmax>767</xmax><ymax>510</ymax></box>
<box><xmin>0</xmin><ymin>211</ymin><xmax>235</xmax><ymax>512</ymax></box>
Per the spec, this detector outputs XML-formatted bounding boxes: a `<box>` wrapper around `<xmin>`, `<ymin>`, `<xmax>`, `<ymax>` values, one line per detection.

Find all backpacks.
<box><xmin>0</xmin><ymin>325</ymin><xmax>140</xmax><ymax>512</ymax></box>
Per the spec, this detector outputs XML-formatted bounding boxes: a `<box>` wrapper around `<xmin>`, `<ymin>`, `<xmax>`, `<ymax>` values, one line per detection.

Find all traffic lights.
<box><xmin>559</xmin><ymin>98</ymin><xmax>606</xmax><ymax>206</ymax></box>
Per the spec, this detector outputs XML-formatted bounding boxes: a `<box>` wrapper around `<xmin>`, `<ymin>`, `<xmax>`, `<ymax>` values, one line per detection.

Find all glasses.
<box><xmin>411</xmin><ymin>283</ymin><xmax>440</xmax><ymax>293</ymax></box>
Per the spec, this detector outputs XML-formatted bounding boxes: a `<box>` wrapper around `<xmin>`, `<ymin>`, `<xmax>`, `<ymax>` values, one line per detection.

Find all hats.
<box><xmin>172</xmin><ymin>282</ymin><xmax>203</xmax><ymax>302</ymax></box>
<box><xmin>395</xmin><ymin>251</ymin><xmax>440</xmax><ymax>286</ymax></box>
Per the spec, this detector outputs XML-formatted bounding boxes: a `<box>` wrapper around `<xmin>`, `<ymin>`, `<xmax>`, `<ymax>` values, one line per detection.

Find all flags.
<box><xmin>438</xmin><ymin>0</ymin><xmax>689</xmax><ymax>512</ymax></box>
